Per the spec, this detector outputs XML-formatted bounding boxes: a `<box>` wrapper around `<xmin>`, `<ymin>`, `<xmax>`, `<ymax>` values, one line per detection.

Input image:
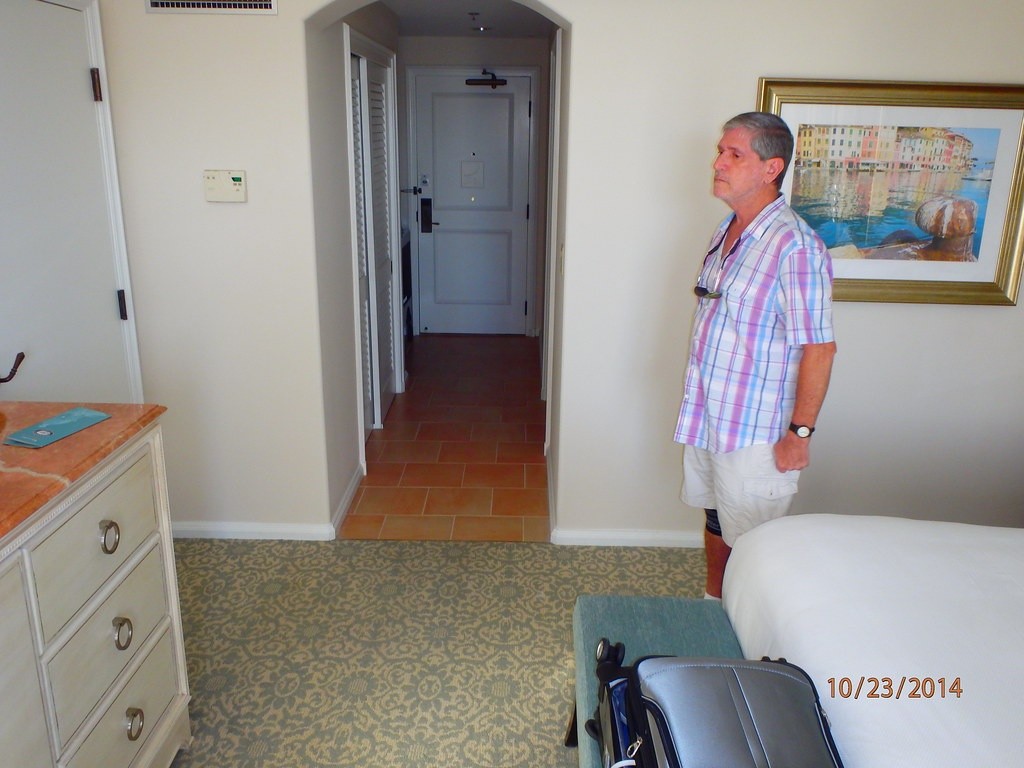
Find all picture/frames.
<box><xmin>757</xmin><ymin>77</ymin><xmax>1023</xmax><ymax>308</ymax></box>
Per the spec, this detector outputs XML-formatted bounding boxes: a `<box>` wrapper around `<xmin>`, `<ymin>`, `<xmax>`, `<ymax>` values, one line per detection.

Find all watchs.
<box><xmin>789</xmin><ymin>422</ymin><xmax>815</xmax><ymax>438</ymax></box>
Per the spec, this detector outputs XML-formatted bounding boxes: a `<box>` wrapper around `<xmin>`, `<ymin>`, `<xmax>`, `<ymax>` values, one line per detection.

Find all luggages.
<box><xmin>585</xmin><ymin>638</ymin><xmax>845</xmax><ymax>768</ymax></box>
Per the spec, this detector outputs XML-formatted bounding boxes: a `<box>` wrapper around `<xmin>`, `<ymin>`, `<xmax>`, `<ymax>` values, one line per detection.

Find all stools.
<box><xmin>561</xmin><ymin>595</ymin><xmax>745</xmax><ymax>767</ymax></box>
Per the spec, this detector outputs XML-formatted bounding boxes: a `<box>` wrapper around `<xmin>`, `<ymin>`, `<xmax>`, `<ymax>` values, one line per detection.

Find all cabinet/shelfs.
<box><xmin>403</xmin><ymin>241</ymin><xmax>415</xmax><ymax>344</ymax></box>
<box><xmin>0</xmin><ymin>426</ymin><xmax>199</xmax><ymax>768</ymax></box>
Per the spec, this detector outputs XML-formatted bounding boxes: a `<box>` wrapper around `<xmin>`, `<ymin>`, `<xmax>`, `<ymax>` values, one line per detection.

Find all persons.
<box><xmin>673</xmin><ymin>112</ymin><xmax>837</xmax><ymax>601</ymax></box>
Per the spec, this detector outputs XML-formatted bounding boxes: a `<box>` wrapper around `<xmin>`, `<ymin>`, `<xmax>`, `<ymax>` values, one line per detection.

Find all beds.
<box><xmin>722</xmin><ymin>514</ymin><xmax>1024</xmax><ymax>768</ymax></box>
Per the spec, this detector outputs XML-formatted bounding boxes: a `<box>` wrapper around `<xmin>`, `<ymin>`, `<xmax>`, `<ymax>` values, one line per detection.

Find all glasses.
<box><xmin>694</xmin><ymin>263</ymin><xmax>723</xmax><ymax>300</ymax></box>
